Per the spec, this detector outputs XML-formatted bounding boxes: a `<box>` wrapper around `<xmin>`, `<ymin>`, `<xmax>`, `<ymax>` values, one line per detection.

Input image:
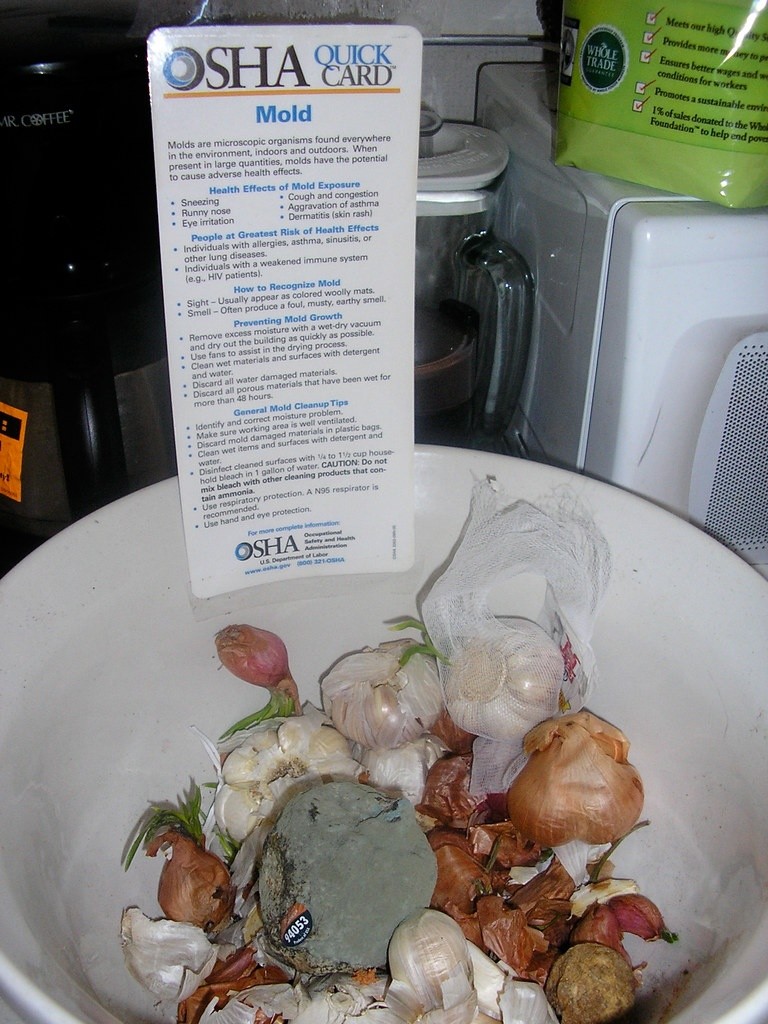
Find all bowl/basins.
<box><xmin>0</xmin><ymin>441</ymin><xmax>766</xmax><ymax>1021</ymax></box>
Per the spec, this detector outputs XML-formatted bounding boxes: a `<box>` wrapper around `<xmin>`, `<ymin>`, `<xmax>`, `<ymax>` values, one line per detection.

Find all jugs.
<box><xmin>417</xmin><ymin>122</ymin><xmax>534</xmax><ymax>444</ymax></box>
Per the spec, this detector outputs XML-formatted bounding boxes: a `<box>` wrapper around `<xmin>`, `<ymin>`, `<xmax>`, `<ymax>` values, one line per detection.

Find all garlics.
<box><xmin>144</xmin><ymin>616</ymin><xmax>681</xmax><ymax>1024</ymax></box>
